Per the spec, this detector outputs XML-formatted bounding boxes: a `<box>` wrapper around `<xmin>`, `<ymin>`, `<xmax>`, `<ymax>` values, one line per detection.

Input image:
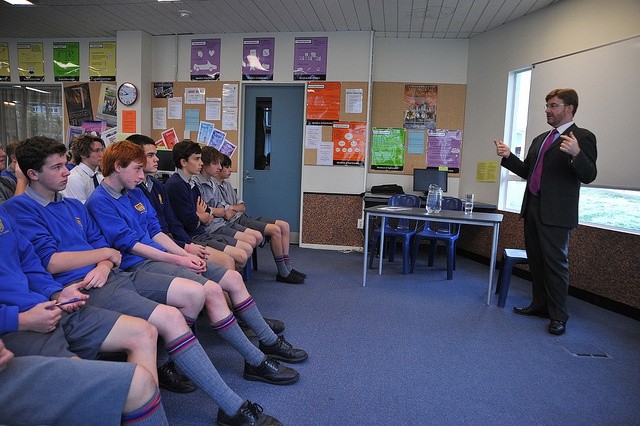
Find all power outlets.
<box><xmin>356</xmin><ymin>218</ymin><xmax>364</xmax><ymax>230</ymax></box>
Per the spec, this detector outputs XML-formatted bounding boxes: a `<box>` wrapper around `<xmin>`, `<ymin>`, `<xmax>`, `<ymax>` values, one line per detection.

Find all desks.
<box><xmin>362</xmin><ymin>205</ymin><xmax>504</xmax><ymax>306</ymax></box>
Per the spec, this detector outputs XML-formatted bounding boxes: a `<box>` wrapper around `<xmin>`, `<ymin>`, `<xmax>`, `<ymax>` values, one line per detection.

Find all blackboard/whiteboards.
<box><xmin>524</xmin><ymin>37</ymin><xmax>640</xmax><ymax>191</ymax></box>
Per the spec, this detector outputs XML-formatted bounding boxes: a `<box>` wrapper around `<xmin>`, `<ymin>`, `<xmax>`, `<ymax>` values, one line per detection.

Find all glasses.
<box><xmin>543</xmin><ymin>102</ymin><xmax>568</xmax><ymax>110</ymax></box>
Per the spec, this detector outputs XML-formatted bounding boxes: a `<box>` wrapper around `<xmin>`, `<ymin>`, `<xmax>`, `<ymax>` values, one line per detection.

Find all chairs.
<box><xmin>409</xmin><ymin>197</ymin><xmax>463</xmax><ymax>280</ymax></box>
<box><xmin>495</xmin><ymin>248</ymin><xmax>528</xmax><ymax>308</ymax></box>
<box><xmin>368</xmin><ymin>194</ymin><xmax>422</xmax><ymax>275</ymax></box>
<box><xmin>243</xmin><ymin>246</ymin><xmax>259</xmax><ymax>284</ymax></box>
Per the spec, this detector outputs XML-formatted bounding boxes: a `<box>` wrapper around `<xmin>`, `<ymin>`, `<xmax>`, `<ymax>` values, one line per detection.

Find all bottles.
<box><xmin>426</xmin><ymin>184</ymin><xmax>443</xmax><ymax>213</ymax></box>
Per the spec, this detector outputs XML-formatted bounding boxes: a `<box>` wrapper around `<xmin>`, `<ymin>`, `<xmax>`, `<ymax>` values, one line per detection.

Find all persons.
<box><xmin>165</xmin><ymin>141</ymin><xmax>253</xmax><ymax>272</ymax></box>
<box><xmin>1</xmin><ymin>142</ymin><xmax>29</xmax><ymax>183</ymax></box>
<box><xmin>0</xmin><ymin>136</ymin><xmax>283</xmax><ymax>426</ymax></box>
<box><xmin>85</xmin><ymin>140</ymin><xmax>308</xmax><ymax>385</ymax></box>
<box><xmin>0</xmin><ymin>339</ymin><xmax>169</xmax><ymax>426</ymax></box>
<box><xmin>493</xmin><ymin>89</ymin><xmax>597</xmax><ymax>336</ymax></box>
<box><xmin>215</xmin><ymin>154</ymin><xmax>306</xmax><ymax>284</ymax></box>
<box><xmin>126</xmin><ymin>133</ymin><xmax>285</xmax><ymax>337</ymax></box>
<box><xmin>59</xmin><ymin>135</ymin><xmax>106</xmax><ymax>205</ymax></box>
<box><xmin>0</xmin><ymin>144</ymin><xmax>29</xmax><ymax>203</ymax></box>
<box><xmin>0</xmin><ymin>204</ymin><xmax>159</xmax><ymax>388</ymax></box>
<box><xmin>192</xmin><ymin>146</ymin><xmax>263</xmax><ymax>249</ymax></box>
<box><xmin>66</xmin><ymin>142</ymin><xmax>76</xmax><ymax>171</ymax></box>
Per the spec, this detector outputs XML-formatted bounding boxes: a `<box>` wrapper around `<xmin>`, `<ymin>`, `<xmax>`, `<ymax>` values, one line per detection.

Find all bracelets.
<box><xmin>187</xmin><ymin>243</ymin><xmax>194</xmax><ymax>253</ymax></box>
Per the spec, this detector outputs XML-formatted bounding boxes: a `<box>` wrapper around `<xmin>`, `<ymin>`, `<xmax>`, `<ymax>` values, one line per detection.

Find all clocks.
<box><xmin>117</xmin><ymin>81</ymin><xmax>138</xmax><ymax>106</ymax></box>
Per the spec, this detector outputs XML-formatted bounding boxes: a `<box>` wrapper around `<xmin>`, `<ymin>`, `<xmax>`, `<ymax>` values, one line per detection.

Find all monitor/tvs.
<box><xmin>412</xmin><ymin>167</ymin><xmax>448</xmax><ymax>194</ymax></box>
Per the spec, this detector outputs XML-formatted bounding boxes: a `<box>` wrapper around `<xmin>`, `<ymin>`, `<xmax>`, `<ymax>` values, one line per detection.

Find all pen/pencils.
<box><xmin>54</xmin><ymin>298</ymin><xmax>81</xmax><ymax>306</ymax></box>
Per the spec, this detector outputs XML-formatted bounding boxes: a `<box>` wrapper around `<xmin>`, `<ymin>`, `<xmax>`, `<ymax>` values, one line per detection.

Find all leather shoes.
<box><xmin>513</xmin><ymin>305</ymin><xmax>550</xmax><ymax>320</ymax></box>
<box><xmin>157</xmin><ymin>361</ymin><xmax>199</xmax><ymax>394</ymax></box>
<box><xmin>276</xmin><ymin>272</ymin><xmax>307</xmax><ymax>284</ymax></box>
<box><xmin>548</xmin><ymin>317</ymin><xmax>565</xmax><ymax>335</ymax></box>
<box><xmin>236</xmin><ymin>316</ymin><xmax>285</xmax><ymax>337</ymax></box>
<box><xmin>259</xmin><ymin>334</ymin><xmax>308</xmax><ymax>364</ymax></box>
<box><xmin>242</xmin><ymin>356</ymin><xmax>300</xmax><ymax>386</ymax></box>
<box><xmin>216</xmin><ymin>398</ymin><xmax>285</xmax><ymax>426</ymax></box>
<box><xmin>292</xmin><ymin>268</ymin><xmax>306</xmax><ymax>278</ymax></box>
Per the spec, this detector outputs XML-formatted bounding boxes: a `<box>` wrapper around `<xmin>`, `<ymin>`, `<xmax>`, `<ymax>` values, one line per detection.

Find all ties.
<box><xmin>93</xmin><ymin>174</ymin><xmax>100</xmax><ymax>188</ymax></box>
<box><xmin>528</xmin><ymin>130</ymin><xmax>560</xmax><ymax>197</ymax></box>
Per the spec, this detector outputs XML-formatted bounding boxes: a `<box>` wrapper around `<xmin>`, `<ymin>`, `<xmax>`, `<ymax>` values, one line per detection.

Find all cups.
<box><xmin>464</xmin><ymin>192</ymin><xmax>475</xmax><ymax>215</ymax></box>
